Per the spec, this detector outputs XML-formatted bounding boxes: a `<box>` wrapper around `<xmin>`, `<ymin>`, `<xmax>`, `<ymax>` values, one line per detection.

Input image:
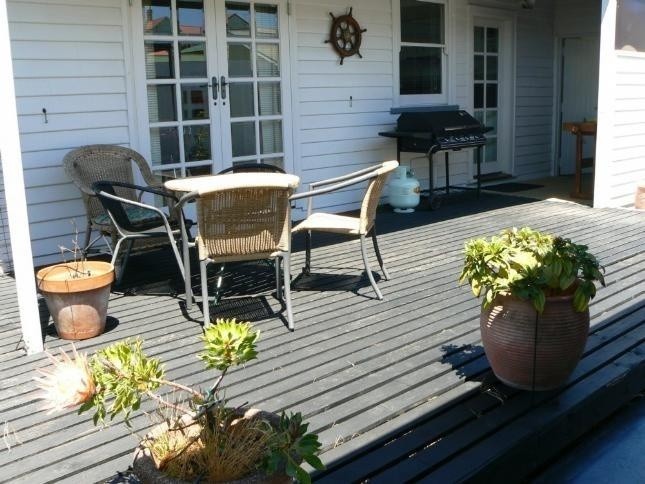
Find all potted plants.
<box><xmin>459</xmin><ymin>227</ymin><xmax>608</xmax><ymax>392</ymax></box>
<box><xmin>36</xmin><ymin>216</ymin><xmax>116</xmax><ymax>340</ymax></box>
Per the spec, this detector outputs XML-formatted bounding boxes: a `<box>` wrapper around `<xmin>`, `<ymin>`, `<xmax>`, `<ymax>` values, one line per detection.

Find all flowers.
<box><xmin>32</xmin><ymin>319</ymin><xmax>326</xmax><ymax>484</ymax></box>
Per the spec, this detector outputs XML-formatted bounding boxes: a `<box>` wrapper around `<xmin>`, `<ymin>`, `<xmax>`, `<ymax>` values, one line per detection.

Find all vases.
<box><xmin>124</xmin><ymin>404</ymin><xmax>304</xmax><ymax>484</ymax></box>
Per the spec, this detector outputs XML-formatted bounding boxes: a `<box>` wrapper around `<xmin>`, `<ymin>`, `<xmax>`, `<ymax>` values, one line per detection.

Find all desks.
<box><xmin>165</xmin><ymin>171</ymin><xmax>301</xmax><ymax>313</ymax></box>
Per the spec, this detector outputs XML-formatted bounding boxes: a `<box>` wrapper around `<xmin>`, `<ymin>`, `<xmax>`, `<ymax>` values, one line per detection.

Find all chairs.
<box><xmin>194</xmin><ymin>183</ymin><xmax>295</xmax><ymax>333</ymax></box>
<box><xmin>288</xmin><ymin>159</ymin><xmax>401</xmax><ymax>300</ymax></box>
<box><xmin>218</xmin><ymin>164</ymin><xmax>285</xmax><ymax>176</ymax></box>
<box><xmin>91</xmin><ymin>179</ymin><xmax>196</xmax><ymax>303</ymax></box>
<box><xmin>63</xmin><ymin>143</ymin><xmax>181</xmax><ymax>281</ymax></box>
